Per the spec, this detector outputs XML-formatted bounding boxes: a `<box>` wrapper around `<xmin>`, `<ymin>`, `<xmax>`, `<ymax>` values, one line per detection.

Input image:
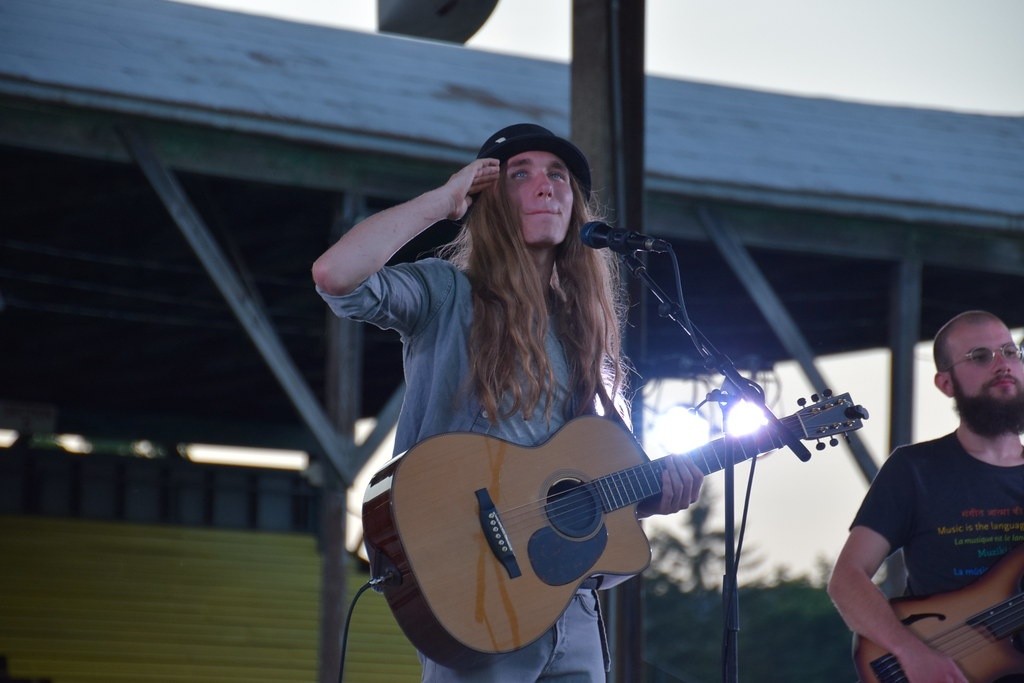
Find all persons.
<box><xmin>830</xmin><ymin>300</ymin><xmax>1023</xmax><ymax>682</ymax></box>
<box><xmin>313</xmin><ymin>124</ymin><xmax>702</xmax><ymax>683</ymax></box>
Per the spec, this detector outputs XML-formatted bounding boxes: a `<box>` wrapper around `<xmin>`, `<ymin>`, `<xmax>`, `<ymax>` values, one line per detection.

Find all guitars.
<box><xmin>851</xmin><ymin>541</ymin><xmax>1024</xmax><ymax>682</ymax></box>
<box><xmin>363</xmin><ymin>389</ymin><xmax>864</xmax><ymax>670</ymax></box>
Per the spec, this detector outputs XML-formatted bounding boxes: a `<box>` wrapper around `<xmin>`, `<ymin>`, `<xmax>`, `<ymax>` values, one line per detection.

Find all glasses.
<box><xmin>945</xmin><ymin>345</ymin><xmax>1023</xmax><ymax>371</ymax></box>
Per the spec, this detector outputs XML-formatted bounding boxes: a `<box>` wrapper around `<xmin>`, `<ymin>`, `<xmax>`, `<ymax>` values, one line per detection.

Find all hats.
<box><xmin>477</xmin><ymin>123</ymin><xmax>593</xmax><ymax>204</ymax></box>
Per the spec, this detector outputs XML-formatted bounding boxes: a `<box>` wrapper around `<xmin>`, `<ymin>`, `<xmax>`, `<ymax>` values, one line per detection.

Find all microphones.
<box><xmin>578</xmin><ymin>220</ymin><xmax>670</xmax><ymax>258</ymax></box>
